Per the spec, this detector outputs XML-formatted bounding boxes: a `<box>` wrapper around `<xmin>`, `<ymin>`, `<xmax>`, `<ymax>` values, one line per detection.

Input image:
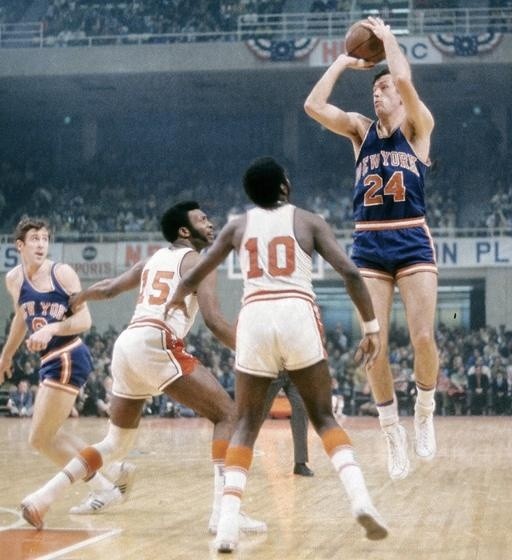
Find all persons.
<box><xmin>0</xmin><ymin>0</ymin><xmax>512</xmax><ymax>48</ymax></box>
<box><xmin>164</xmin><ymin>157</ymin><xmax>389</xmax><ymax>553</ymax></box>
<box><xmin>303</xmin><ymin>15</ymin><xmax>440</xmax><ymax>482</ymax></box>
<box><xmin>2</xmin><ymin>319</ymin><xmax>511</xmax><ymax>416</ymax></box>
<box><xmin>0</xmin><ymin>217</ymin><xmax>138</xmax><ymax>515</ymax></box>
<box><xmin>18</xmin><ymin>201</ymin><xmax>268</xmax><ymax>533</ymax></box>
<box><xmin>0</xmin><ymin>67</ymin><xmax>512</xmax><ymax>233</ymax></box>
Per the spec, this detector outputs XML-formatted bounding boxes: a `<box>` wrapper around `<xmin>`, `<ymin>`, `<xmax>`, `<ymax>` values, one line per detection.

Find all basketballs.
<box><xmin>344</xmin><ymin>18</ymin><xmax>386</xmax><ymax>63</ymax></box>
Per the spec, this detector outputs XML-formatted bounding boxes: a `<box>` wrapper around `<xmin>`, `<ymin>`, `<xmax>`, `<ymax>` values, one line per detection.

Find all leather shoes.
<box><xmin>294</xmin><ymin>465</ymin><xmax>314</xmax><ymax>476</ymax></box>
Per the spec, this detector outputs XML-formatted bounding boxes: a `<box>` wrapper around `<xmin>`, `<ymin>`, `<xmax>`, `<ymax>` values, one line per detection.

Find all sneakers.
<box><xmin>21</xmin><ymin>489</ymin><xmax>51</xmax><ymax>529</ymax></box>
<box><xmin>355</xmin><ymin>497</ymin><xmax>388</xmax><ymax>544</ymax></box>
<box><xmin>378</xmin><ymin>414</ymin><xmax>435</xmax><ymax>481</ymax></box>
<box><xmin>209</xmin><ymin>507</ymin><xmax>267</xmax><ymax>552</ymax></box>
<box><xmin>69</xmin><ymin>462</ymin><xmax>137</xmax><ymax>514</ymax></box>
